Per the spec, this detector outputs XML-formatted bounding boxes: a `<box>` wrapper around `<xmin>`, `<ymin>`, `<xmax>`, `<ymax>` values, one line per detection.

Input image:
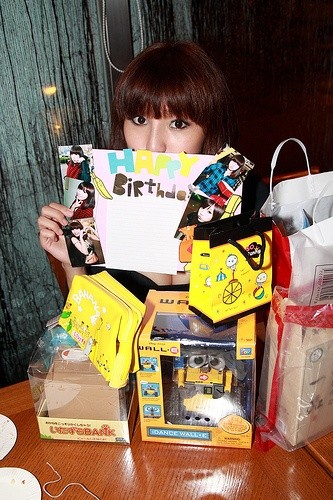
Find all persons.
<box><xmin>38</xmin><ymin>40</ymin><xmax>271</xmax><ymax>306</ymax></box>
<box><xmin>57</xmin><ymin>145</ymin><xmax>93</xmax><ymax>183</ymax></box>
<box><xmin>60</xmin><ymin>220</ymin><xmax>102</xmax><ymax>266</ymax></box>
<box><xmin>64</xmin><ymin>181</ymin><xmax>94</xmax><ymax>220</ymax></box>
<box><xmin>193</xmin><ymin>154</ymin><xmax>245</xmax><ymax>203</ymax></box>
<box><xmin>179</xmin><ymin>196</ymin><xmax>225</xmax><ymax>243</ymax></box>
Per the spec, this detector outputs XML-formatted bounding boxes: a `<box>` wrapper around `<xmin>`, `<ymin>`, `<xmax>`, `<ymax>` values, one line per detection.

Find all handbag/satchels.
<box><xmin>57</xmin><ymin>270</ymin><xmax>146</xmax><ymax>388</ymax></box>
<box><xmin>248</xmin><ymin>283</ymin><xmax>333</xmax><ymax>449</ymax></box>
<box><xmin>188</xmin><ymin>210</ymin><xmax>273</xmax><ymax>327</ymax></box>
<box><xmin>261</xmin><ymin>138</ymin><xmax>332</xmax><ymax>324</ymax></box>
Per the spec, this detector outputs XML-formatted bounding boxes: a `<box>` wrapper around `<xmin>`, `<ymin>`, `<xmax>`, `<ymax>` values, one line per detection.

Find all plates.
<box><xmin>0</xmin><ymin>413</ymin><xmax>17</xmax><ymax>461</ymax></box>
<box><xmin>0</xmin><ymin>467</ymin><xmax>41</xmax><ymax>500</ymax></box>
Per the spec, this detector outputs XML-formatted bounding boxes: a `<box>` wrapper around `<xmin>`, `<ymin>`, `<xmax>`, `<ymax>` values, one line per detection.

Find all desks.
<box><xmin>1</xmin><ymin>379</ymin><xmax>333</xmax><ymax>500</ymax></box>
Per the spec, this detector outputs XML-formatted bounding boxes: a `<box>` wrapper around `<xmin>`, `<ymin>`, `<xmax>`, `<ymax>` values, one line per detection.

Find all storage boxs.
<box><xmin>27</xmin><ymin>322</ymin><xmax>137</xmax><ymax>443</ymax></box>
<box><xmin>264</xmin><ymin>297</ymin><xmax>333</xmax><ymax>446</ymax></box>
<box><xmin>136</xmin><ymin>289</ymin><xmax>257</xmax><ymax>450</ymax></box>
